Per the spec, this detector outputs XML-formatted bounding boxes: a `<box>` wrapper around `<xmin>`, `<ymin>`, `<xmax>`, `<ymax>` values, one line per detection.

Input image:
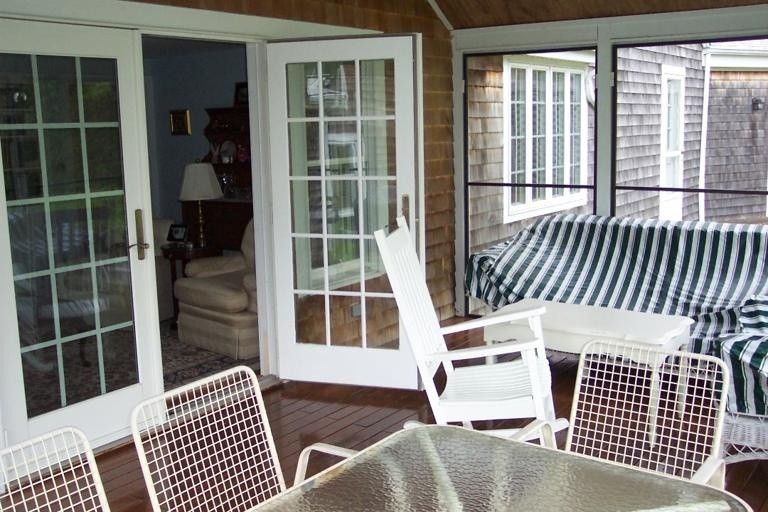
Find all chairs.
<box><xmin>374</xmin><ymin>214</ymin><xmax>568</xmax><ymax>452</ymax></box>
<box><xmin>172</xmin><ymin>218</ymin><xmax>260</xmax><ymax>359</ymax></box>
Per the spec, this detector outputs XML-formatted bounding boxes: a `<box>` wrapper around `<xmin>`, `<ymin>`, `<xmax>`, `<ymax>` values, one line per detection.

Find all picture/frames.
<box><xmin>169</xmin><ymin>108</ymin><xmax>192</xmax><ymax>136</ymax></box>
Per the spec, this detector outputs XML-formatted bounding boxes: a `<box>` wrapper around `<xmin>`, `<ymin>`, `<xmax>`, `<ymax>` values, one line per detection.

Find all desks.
<box><xmin>160</xmin><ymin>240</ymin><xmax>224</xmax><ymax>320</ymax></box>
<box><xmin>479</xmin><ymin>297</ymin><xmax>694</xmax><ymax>447</ymax></box>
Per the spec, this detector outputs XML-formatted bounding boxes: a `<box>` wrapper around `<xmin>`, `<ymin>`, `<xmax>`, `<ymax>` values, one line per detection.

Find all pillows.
<box><xmin>150</xmin><ymin>217</ymin><xmax>172</xmax><ymax>257</ymax></box>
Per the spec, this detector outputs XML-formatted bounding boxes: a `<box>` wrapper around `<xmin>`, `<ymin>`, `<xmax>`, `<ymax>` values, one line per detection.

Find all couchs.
<box><xmin>470</xmin><ymin>215</ymin><xmax>768</xmax><ymax>416</ymax></box>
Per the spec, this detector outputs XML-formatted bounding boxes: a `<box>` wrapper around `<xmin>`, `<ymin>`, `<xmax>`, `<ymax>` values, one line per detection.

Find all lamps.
<box><xmin>177</xmin><ymin>163</ymin><xmax>224</xmax><ymax>248</ymax></box>
